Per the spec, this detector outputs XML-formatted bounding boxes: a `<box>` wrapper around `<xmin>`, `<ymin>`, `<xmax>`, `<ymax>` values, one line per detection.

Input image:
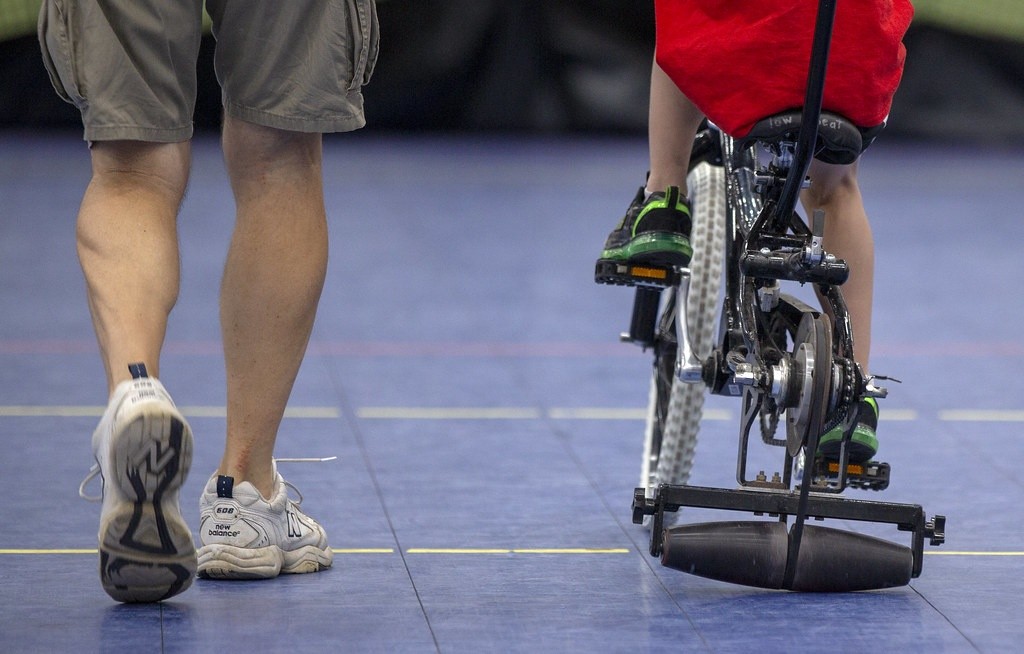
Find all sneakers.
<box><xmin>602</xmin><ymin>173</ymin><xmax>693</xmax><ymax>266</ymax></box>
<box><xmin>79</xmin><ymin>363</ymin><xmax>197</xmax><ymax>602</ymax></box>
<box><xmin>199</xmin><ymin>455</ymin><xmax>336</xmax><ymax>578</ymax></box>
<box><xmin>818</xmin><ymin>393</ymin><xmax>879</xmax><ymax>463</ymax></box>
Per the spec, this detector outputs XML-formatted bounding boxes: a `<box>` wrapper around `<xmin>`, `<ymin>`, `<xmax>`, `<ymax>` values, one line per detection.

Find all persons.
<box><xmin>603</xmin><ymin>0</ymin><xmax>915</xmax><ymax>464</ymax></box>
<box><xmin>36</xmin><ymin>0</ymin><xmax>380</xmax><ymax>603</ymax></box>
